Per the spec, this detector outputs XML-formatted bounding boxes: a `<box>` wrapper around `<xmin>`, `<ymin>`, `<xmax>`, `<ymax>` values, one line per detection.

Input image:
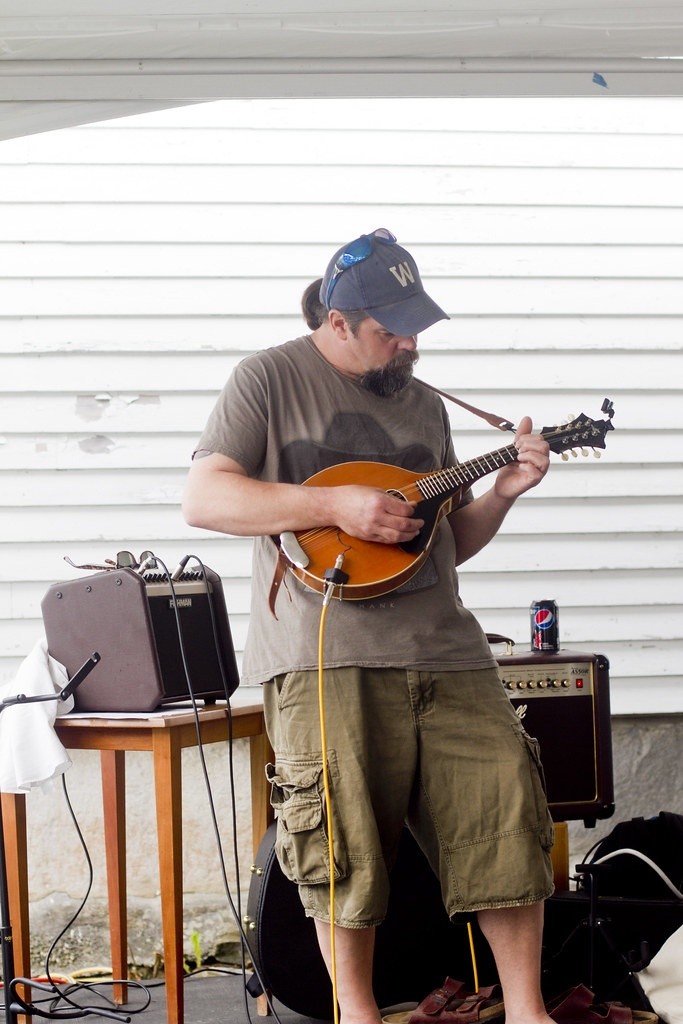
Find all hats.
<box><xmin>319</xmin><ymin>235</ymin><xmax>451</xmax><ymax>338</ymax></box>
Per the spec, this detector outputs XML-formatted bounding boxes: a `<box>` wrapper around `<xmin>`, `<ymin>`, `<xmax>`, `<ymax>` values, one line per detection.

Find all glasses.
<box><xmin>325</xmin><ymin>228</ymin><xmax>397</xmax><ymax>311</ymax></box>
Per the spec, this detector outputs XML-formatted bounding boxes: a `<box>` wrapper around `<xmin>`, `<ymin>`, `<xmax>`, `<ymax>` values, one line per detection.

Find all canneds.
<box><xmin>529</xmin><ymin>599</ymin><xmax>560</xmax><ymax>654</ymax></box>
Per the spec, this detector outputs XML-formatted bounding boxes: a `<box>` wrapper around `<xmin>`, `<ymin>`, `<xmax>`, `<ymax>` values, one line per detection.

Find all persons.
<box><xmin>180</xmin><ymin>228</ymin><xmax>556</xmax><ymax>1024</ymax></box>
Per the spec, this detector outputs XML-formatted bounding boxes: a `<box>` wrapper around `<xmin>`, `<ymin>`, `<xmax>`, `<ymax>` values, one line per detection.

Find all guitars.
<box><xmin>278</xmin><ymin>411</ymin><xmax>617</xmax><ymax>604</ymax></box>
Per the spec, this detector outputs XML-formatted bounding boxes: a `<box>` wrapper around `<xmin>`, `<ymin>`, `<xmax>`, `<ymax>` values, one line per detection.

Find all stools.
<box><xmin>550</xmin><ymin>821</ymin><xmax>568</xmax><ymax>891</ymax></box>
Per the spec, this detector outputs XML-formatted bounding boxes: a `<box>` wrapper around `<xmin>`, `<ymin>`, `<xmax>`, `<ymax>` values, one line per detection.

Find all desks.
<box><xmin>0</xmin><ymin>685</ymin><xmax>285</xmax><ymax>1023</ymax></box>
